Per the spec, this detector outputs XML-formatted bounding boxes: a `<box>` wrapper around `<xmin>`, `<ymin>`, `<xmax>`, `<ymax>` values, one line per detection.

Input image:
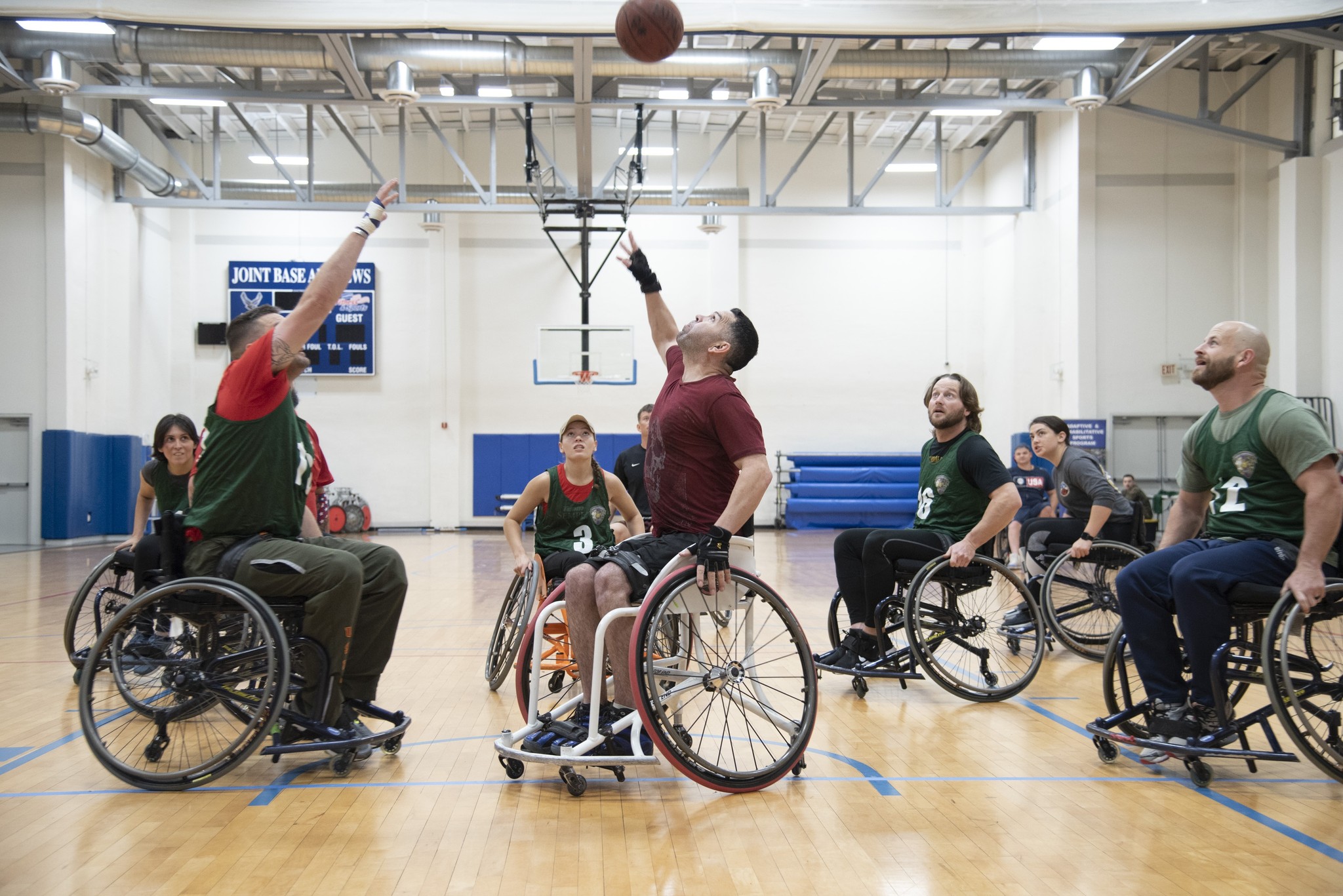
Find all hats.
<box><xmin>559</xmin><ymin>415</ymin><xmax>595</xmax><ymax>441</ymax></box>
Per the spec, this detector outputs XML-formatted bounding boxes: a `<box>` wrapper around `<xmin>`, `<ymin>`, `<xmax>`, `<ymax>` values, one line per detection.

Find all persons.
<box><xmin>115</xmin><ymin>178</ymin><xmax>410</xmax><ymax>758</ymax></box>
<box><xmin>1001</xmin><ymin>415</ymin><xmax>1153</xmax><ymax>631</ymax></box>
<box><xmin>502</xmin><ymin>229</ymin><xmax>773</xmax><ymax>755</ymax></box>
<box><xmin>819</xmin><ymin>374</ymin><xmax>1022</xmax><ymax>670</ymax></box>
<box><xmin>1115</xmin><ymin>321</ymin><xmax>1343</xmax><ymax>766</ymax></box>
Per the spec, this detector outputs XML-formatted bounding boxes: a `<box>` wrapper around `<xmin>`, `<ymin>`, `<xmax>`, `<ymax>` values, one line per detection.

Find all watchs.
<box><xmin>1080</xmin><ymin>532</ymin><xmax>1095</xmax><ymax>541</ymax></box>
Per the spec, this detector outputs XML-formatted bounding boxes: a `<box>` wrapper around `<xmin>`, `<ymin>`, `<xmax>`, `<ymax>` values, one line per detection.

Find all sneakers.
<box><xmin>523</xmin><ymin>700</ymin><xmax>614</xmax><ymax>756</ymax></box>
<box><xmin>287</xmin><ymin>687</ymin><xmax>385</xmax><ymax>759</ymax></box>
<box><xmin>1002</xmin><ymin>601</ymin><xmax>1057</xmax><ymax>628</ymax></box>
<box><xmin>1140</xmin><ymin>697</ymin><xmax>1190</xmax><ymax>764</ymax></box>
<box><xmin>819</xmin><ymin>627</ymin><xmax>897</xmax><ymax>674</ymax></box>
<box><xmin>134</xmin><ymin>632</ymin><xmax>174</xmax><ymax>678</ymax></box>
<box><xmin>120</xmin><ymin>631</ymin><xmax>155</xmax><ymax>673</ymax></box>
<box><xmin>1164</xmin><ymin>696</ymin><xmax>1238</xmax><ymax>759</ymax></box>
<box><xmin>550</xmin><ymin>698</ymin><xmax>654</xmax><ymax>757</ymax></box>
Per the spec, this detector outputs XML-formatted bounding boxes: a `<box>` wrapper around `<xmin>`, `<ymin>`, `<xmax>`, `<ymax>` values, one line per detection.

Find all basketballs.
<box><xmin>615</xmin><ymin>0</ymin><xmax>685</xmax><ymax>64</ymax></box>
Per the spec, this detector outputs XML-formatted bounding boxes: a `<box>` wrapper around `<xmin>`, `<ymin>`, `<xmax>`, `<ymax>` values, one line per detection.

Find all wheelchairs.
<box><xmin>996</xmin><ymin>541</ymin><xmax>1153</xmax><ymax>664</ymax></box>
<box><xmin>63</xmin><ymin>543</ymin><xmax>413</xmax><ymax>792</ymax></box>
<box><xmin>484</xmin><ymin>532</ymin><xmax>815</xmax><ymax>795</ymax></box>
<box><xmin>1085</xmin><ymin>578</ymin><xmax>1343</xmax><ymax>788</ymax></box>
<box><xmin>813</xmin><ymin>529</ymin><xmax>1045</xmax><ymax>703</ymax></box>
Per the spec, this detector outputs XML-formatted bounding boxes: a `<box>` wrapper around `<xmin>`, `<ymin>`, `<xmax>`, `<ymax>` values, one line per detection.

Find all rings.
<box><xmin>1315</xmin><ymin>595</ymin><xmax>1321</xmax><ymax>600</ymax></box>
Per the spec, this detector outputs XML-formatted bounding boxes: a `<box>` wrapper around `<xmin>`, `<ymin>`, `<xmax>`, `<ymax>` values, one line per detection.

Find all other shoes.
<box><xmin>1007</xmin><ymin>553</ymin><xmax>1023</xmax><ymax>569</ymax></box>
